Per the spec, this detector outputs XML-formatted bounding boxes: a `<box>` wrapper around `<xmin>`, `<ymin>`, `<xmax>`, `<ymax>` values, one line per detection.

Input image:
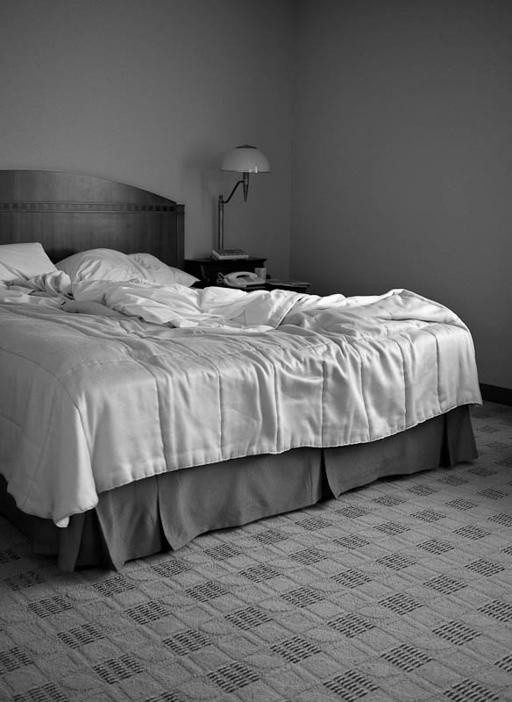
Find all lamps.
<box><xmin>211</xmin><ymin>143</ymin><xmax>272</xmax><ymax>259</ymax></box>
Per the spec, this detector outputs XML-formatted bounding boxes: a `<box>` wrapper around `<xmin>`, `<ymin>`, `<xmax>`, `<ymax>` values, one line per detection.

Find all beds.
<box><xmin>0</xmin><ymin>168</ymin><xmax>484</xmax><ymax>571</ymax></box>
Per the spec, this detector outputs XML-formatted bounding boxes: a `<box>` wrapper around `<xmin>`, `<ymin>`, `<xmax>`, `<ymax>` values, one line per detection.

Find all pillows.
<box><xmin>0</xmin><ymin>242</ymin><xmax>57</xmax><ymax>279</ymax></box>
<box><xmin>53</xmin><ymin>246</ymin><xmax>202</xmax><ymax>287</ymax></box>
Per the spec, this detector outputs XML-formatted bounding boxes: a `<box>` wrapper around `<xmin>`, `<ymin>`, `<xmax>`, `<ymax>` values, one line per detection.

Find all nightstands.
<box><xmin>183</xmin><ymin>258</ymin><xmax>267</xmax><ymax>289</ymax></box>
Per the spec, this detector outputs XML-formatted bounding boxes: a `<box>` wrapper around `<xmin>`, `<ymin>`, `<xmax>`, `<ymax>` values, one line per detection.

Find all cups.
<box><xmin>255</xmin><ymin>267</ymin><xmax>267</xmax><ymax>281</ymax></box>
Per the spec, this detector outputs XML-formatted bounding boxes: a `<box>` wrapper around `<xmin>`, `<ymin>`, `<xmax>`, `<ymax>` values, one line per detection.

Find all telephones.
<box><xmin>217</xmin><ymin>271</ymin><xmax>265</xmax><ymax>288</ymax></box>
